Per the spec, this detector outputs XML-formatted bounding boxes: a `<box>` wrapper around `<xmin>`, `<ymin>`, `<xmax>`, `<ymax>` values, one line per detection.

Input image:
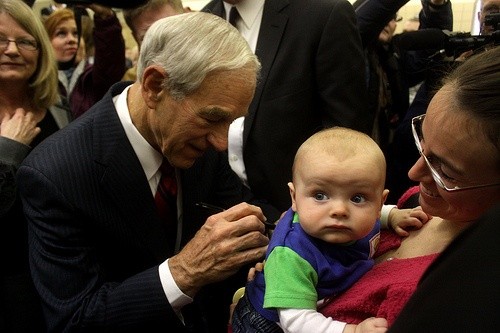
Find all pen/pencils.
<box><xmin>194</xmin><ymin>202</ymin><xmax>277</xmax><ymax>230</ymax></box>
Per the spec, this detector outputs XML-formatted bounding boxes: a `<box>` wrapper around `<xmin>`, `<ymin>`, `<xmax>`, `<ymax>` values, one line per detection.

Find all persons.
<box><xmin>249</xmin><ymin>46</ymin><xmax>500</xmax><ymax>333</ymax></box>
<box><xmin>233</xmin><ymin>127</ymin><xmax>432</xmax><ymax>333</ymax></box>
<box><xmin>0</xmin><ymin>0</ymin><xmax>72</xmax><ymax>333</ymax></box>
<box><xmin>17</xmin><ymin>11</ymin><xmax>288</xmax><ymax>333</ymax></box>
<box><xmin>32</xmin><ymin>0</ymin><xmax>500</xmax><ymax>205</ymax></box>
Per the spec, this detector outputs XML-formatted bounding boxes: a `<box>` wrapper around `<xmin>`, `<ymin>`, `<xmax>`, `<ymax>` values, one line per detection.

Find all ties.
<box><xmin>229</xmin><ymin>7</ymin><xmax>238</xmax><ymax>26</ymax></box>
<box><xmin>154</xmin><ymin>157</ymin><xmax>178</xmax><ymax>237</ymax></box>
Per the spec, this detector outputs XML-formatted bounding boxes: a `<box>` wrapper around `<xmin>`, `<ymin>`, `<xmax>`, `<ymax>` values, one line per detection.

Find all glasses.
<box><xmin>0</xmin><ymin>33</ymin><xmax>40</xmax><ymax>50</ymax></box>
<box><xmin>412</xmin><ymin>114</ymin><xmax>500</xmax><ymax>193</ymax></box>
